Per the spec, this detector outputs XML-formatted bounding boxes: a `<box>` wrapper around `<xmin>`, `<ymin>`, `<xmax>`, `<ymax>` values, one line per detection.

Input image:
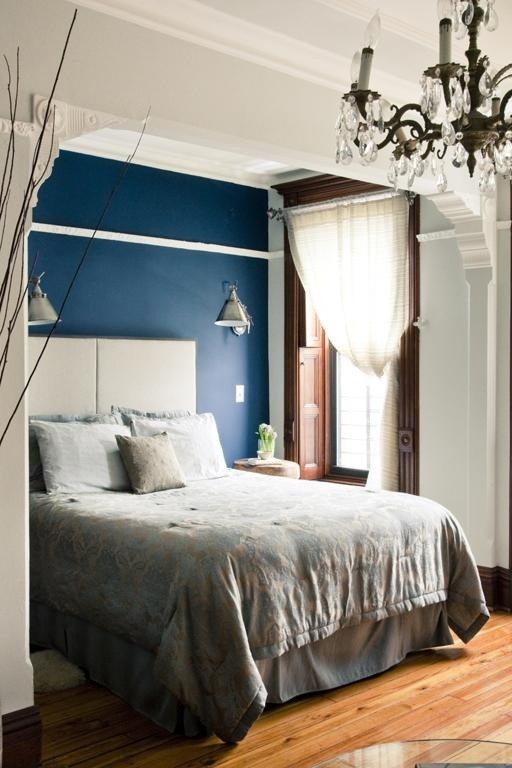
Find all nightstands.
<box><xmin>232</xmin><ymin>456</ymin><xmax>300</xmax><ymax>480</ymax></box>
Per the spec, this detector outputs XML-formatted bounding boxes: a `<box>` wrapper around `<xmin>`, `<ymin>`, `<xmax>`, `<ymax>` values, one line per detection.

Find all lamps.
<box><xmin>28</xmin><ymin>271</ymin><xmax>62</xmax><ymax>325</ymax></box>
<box><xmin>214</xmin><ymin>279</ymin><xmax>254</xmax><ymax>337</ymax></box>
<box><xmin>334</xmin><ymin>0</ymin><xmax>512</xmax><ymax>202</ymax></box>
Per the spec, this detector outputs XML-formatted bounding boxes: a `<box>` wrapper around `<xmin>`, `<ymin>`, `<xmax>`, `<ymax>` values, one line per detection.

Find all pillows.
<box><xmin>26</xmin><ymin>403</ymin><xmax>229</xmax><ymax>496</ymax></box>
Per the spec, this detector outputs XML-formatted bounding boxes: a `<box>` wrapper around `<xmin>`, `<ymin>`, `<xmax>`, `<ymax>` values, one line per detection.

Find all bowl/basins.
<box><xmin>257</xmin><ymin>450</ymin><xmax>273</xmax><ymax>460</ymax></box>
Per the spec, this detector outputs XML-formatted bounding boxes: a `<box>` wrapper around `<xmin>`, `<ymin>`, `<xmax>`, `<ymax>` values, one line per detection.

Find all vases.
<box><xmin>257</xmin><ymin>439</ymin><xmax>275</xmax><ymax>459</ymax></box>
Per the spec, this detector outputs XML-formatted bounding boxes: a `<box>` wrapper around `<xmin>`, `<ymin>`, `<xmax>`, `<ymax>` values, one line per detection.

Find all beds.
<box><xmin>26</xmin><ymin>334</ymin><xmax>459</xmax><ymax>738</ymax></box>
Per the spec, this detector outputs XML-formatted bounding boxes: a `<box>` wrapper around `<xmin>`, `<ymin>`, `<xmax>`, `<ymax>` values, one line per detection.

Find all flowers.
<box><xmin>254</xmin><ymin>422</ymin><xmax>277</xmax><ymax>451</ymax></box>
